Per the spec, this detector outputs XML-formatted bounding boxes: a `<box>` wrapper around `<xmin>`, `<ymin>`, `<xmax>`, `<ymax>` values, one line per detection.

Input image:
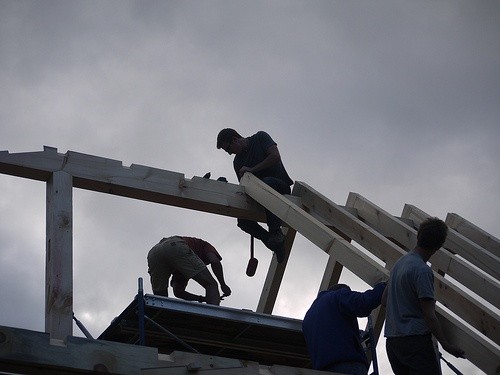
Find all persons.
<box><xmin>146</xmin><ymin>236</ymin><xmax>232</xmax><ymax>306</ymax></box>
<box><xmin>302</xmin><ymin>280</ymin><xmax>387</xmax><ymax>375</ymax></box>
<box><xmin>384</xmin><ymin>218</ymin><xmax>463</xmax><ymax>375</ymax></box>
<box><xmin>216</xmin><ymin>128</ymin><xmax>294</xmax><ymax>264</ymax></box>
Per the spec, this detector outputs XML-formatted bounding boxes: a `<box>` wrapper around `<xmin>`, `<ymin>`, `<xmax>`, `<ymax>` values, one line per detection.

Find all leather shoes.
<box><xmin>268</xmin><ymin>215</ymin><xmax>286</xmax><ymax>244</ymax></box>
<box><xmin>263</xmin><ymin>234</ymin><xmax>286</xmax><ymax>264</ymax></box>
